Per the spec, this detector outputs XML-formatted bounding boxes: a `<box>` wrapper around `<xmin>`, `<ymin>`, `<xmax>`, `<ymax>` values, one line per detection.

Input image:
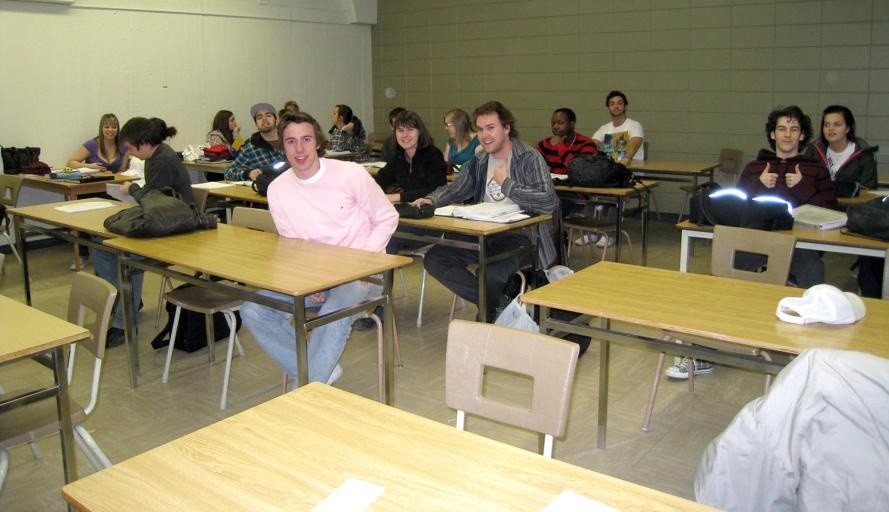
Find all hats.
<box><xmin>250</xmin><ymin>103</ymin><xmax>276</xmax><ymax>118</ymax></box>
<box><xmin>775</xmin><ymin>284</ymin><xmax>866</xmax><ymax>326</ymax></box>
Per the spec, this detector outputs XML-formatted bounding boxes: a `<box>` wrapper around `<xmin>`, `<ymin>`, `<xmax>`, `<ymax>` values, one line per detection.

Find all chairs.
<box><xmin>562</xmin><ymin>191</ymin><xmax>638</xmax><ymax>266</ymax></box>
<box><xmin>0</xmin><ymin>271</ymin><xmax>118</xmax><ymax>494</ymax></box>
<box><xmin>447</xmin><ymin>189</ymin><xmax>558</xmax><ymax>324</ymax></box>
<box><xmin>641</xmin><ymin>222</ymin><xmax>796</xmax><ymax>432</ymax></box>
<box><xmin>162</xmin><ymin>206</ymin><xmax>277</xmax><ymax>409</ymax></box>
<box><xmin>591</xmin><ymin>139</ymin><xmax>665</xmax><ymax>221</ymax></box>
<box><xmin>1</xmin><ymin>173</ymin><xmax>24</xmax><ymax>273</ymax></box>
<box><xmin>445</xmin><ymin>319</ymin><xmax>580</xmax><ymax>458</ymax></box>
<box><xmin>266</xmin><ymin>241</ymin><xmax>404</xmax><ymax>404</ymax></box>
<box><xmin>384</xmin><ymin>175</ymin><xmax>463</xmax><ymax>325</ymax></box>
<box><xmin>680</xmin><ymin>145</ymin><xmax>745</xmax><ymax>222</ymax></box>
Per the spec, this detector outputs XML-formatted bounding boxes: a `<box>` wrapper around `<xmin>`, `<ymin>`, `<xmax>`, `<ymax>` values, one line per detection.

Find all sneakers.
<box><xmin>665</xmin><ymin>356</ymin><xmax>714</xmax><ymax>378</ymax></box>
<box><xmin>575</xmin><ymin>234</ymin><xmax>597</xmax><ymax>245</ymax></box>
<box><xmin>354</xmin><ymin>306</ymin><xmax>384</xmax><ymax>330</ymax></box>
<box><xmin>105</xmin><ymin>327</ymin><xmax>137</xmax><ymax>348</ymax></box>
<box><xmin>596</xmin><ymin>236</ymin><xmax>616</xmax><ymax>247</ymax></box>
<box><xmin>70</xmin><ymin>255</ymin><xmax>88</xmax><ymax>270</ymax></box>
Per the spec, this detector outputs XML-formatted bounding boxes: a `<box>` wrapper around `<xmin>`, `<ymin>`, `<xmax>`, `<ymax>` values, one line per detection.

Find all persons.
<box><xmin>806</xmin><ymin>107</ymin><xmax>878</xmax><ymax>298</ymax></box>
<box><xmin>224</xmin><ymin>103</ymin><xmax>294</xmax><ymax>181</ymax></box>
<box><xmin>574</xmin><ymin>90</ymin><xmax>644</xmax><ymax>248</ymax></box>
<box><xmin>88</xmin><ymin>116</ymin><xmax>198</xmax><ymax>349</ymax></box>
<box><xmin>122</xmin><ymin>118</ymin><xmax>178</xmax><ymax>187</ymax></box>
<box><xmin>443</xmin><ymin>108</ymin><xmax>484</xmax><ymax>164</ymax></box>
<box><xmin>534</xmin><ymin>107</ymin><xmax>598</xmax><ymax>245</ymax></box>
<box><xmin>329</xmin><ymin>104</ymin><xmax>369</xmax><ymax>163</ymax></box>
<box><xmin>381</xmin><ymin>108</ymin><xmax>411</xmax><ymax>160</ymax></box>
<box><xmin>278</xmin><ymin>100</ymin><xmax>301</xmax><ymax>116</ymax></box>
<box><xmin>240</xmin><ymin>111</ymin><xmax>399</xmax><ymax>391</ymax></box>
<box><xmin>207</xmin><ymin>110</ymin><xmax>242</xmax><ymax>148</ymax></box>
<box><xmin>352</xmin><ymin>111</ymin><xmax>447</xmax><ymax>331</ymax></box>
<box><xmin>663</xmin><ymin>105</ymin><xmax>839</xmax><ymax>379</ymax></box>
<box><xmin>66</xmin><ymin>113</ymin><xmax>129</xmax><ymax>271</ymax></box>
<box><xmin>407</xmin><ymin>100</ymin><xmax>559</xmax><ymax>323</ymax></box>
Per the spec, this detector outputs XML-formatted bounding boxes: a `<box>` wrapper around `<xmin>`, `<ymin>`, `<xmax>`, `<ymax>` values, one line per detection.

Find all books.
<box><xmin>106</xmin><ymin>183</ymin><xmax>137</xmax><ymax>204</ymax></box>
<box><xmin>191</xmin><ymin>179</ymin><xmax>258</xmax><ymax>191</ymax></box>
<box><xmin>53</xmin><ymin>202</ymin><xmax>117</xmax><ymax>213</ymax></box>
<box><xmin>434</xmin><ymin>201</ymin><xmax>531</xmax><ymax>224</ymax></box>
<box><xmin>787</xmin><ymin>204</ymin><xmax>849</xmax><ymax>231</ymax></box>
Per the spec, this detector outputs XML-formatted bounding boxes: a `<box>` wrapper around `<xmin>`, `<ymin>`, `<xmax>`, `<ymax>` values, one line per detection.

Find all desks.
<box><xmin>832</xmin><ymin>186</ymin><xmax>889</xmax><ymax>204</ymax></box>
<box><xmin>20</xmin><ymin>171</ymin><xmax>149</xmax><ymax>272</ymax></box>
<box><xmin>548</xmin><ymin>175</ymin><xmax>658</xmax><ymax>265</ymax></box>
<box><xmin>61</xmin><ymin>380</ymin><xmax>732</xmax><ymax>512</ymax></box>
<box><xmin>0</xmin><ymin>292</ymin><xmax>88</xmax><ymax>512</ymax></box>
<box><xmin>179</xmin><ymin>149</ymin><xmax>245</xmax><ymax>224</ymax></box>
<box><xmin>398</xmin><ymin>205</ymin><xmax>550</xmax><ymax>321</ymax></box>
<box><xmin>625</xmin><ymin>145</ymin><xmax>719</xmax><ymax>224</ymax></box>
<box><xmin>518</xmin><ymin>258</ymin><xmax>889</xmax><ymax>362</ymax></box>
<box><xmin>319</xmin><ymin>143</ymin><xmax>357</xmax><ymax>162</ymax></box>
<box><xmin>6</xmin><ymin>198</ymin><xmax>141</xmax><ymax>378</ymax></box>
<box><xmin>365</xmin><ymin>140</ymin><xmax>386</xmax><ymax>163</ymax></box>
<box><xmin>675</xmin><ymin>220</ymin><xmax>889</xmax><ymax>300</ymax></box>
<box><xmin>103</xmin><ymin>222</ymin><xmax>411</xmax><ymax>405</ymax></box>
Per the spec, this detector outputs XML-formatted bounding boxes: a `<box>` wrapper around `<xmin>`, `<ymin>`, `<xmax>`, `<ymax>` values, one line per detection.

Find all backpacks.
<box><xmin>252</xmin><ymin>171</ymin><xmax>279</xmax><ymax>197</ymax></box>
<box><xmin>688</xmin><ymin>182</ymin><xmax>794</xmax><ymax>231</ymax></box>
<box><xmin>151</xmin><ymin>282</ymin><xmax>241</xmax><ymax>354</ymax></box>
<box><xmin>552</xmin><ymin>151</ymin><xmax>635</xmax><ymax>188</ymax></box>
<box><xmin>840</xmin><ymin>196</ymin><xmax>889</xmax><ymax>242</ymax></box>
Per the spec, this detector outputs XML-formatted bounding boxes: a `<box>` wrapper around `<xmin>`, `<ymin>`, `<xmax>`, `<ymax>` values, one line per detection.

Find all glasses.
<box><xmin>445</xmin><ymin>122</ymin><xmax>453</xmax><ymax>127</ymax></box>
